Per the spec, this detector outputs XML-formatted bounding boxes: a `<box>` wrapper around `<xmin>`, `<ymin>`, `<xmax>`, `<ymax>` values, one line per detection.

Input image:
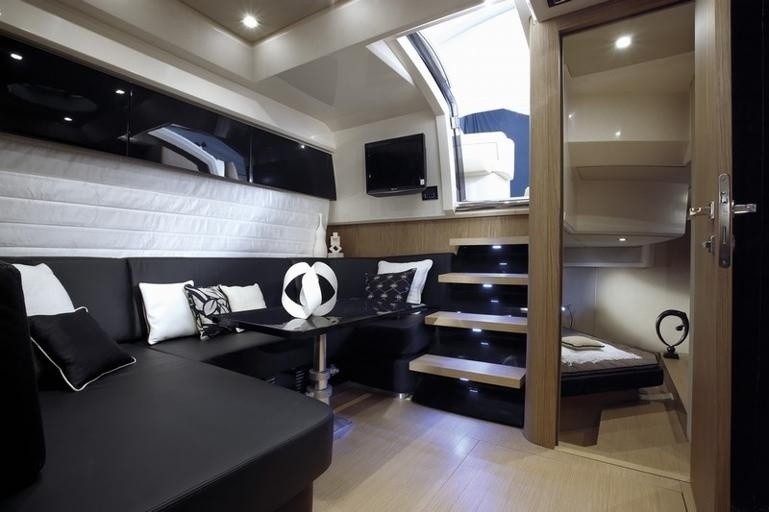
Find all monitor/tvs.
<box><xmin>365</xmin><ymin>133</ymin><xmax>425</xmax><ymax>195</ymax></box>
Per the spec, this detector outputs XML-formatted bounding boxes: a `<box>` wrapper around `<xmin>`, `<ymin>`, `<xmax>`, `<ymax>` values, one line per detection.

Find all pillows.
<box><xmin>138</xmin><ymin>278</ymin><xmax>267</xmax><ymax>346</ymax></box>
<box><xmin>561</xmin><ymin>336</ymin><xmax>605</xmax><ymax>350</ymax></box>
<box><xmin>363</xmin><ymin>259</ymin><xmax>433</xmax><ymax>321</ymax></box>
<box><xmin>10</xmin><ymin>263</ymin><xmax>136</xmax><ymax>392</ymax></box>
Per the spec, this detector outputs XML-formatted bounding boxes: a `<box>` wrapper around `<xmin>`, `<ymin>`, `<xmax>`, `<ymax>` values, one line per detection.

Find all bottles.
<box><xmin>313</xmin><ymin>213</ymin><xmax>328</xmax><ymax>257</ymax></box>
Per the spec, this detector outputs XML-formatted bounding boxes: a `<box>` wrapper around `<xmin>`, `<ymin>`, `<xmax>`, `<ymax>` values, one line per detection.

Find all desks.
<box><xmin>213</xmin><ymin>301</ymin><xmax>427</xmax><ymax>440</ymax></box>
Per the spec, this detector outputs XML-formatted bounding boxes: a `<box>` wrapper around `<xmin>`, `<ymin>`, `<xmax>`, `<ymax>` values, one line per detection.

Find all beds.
<box><xmin>561</xmin><ymin>327</ymin><xmax>664</xmax><ymax>396</ymax></box>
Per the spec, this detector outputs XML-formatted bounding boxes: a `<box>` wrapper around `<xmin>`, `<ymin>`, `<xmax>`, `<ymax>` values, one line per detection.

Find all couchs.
<box><xmin>0</xmin><ymin>253</ymin><xmax>456</xmax><ymax>512</ymax></box>
